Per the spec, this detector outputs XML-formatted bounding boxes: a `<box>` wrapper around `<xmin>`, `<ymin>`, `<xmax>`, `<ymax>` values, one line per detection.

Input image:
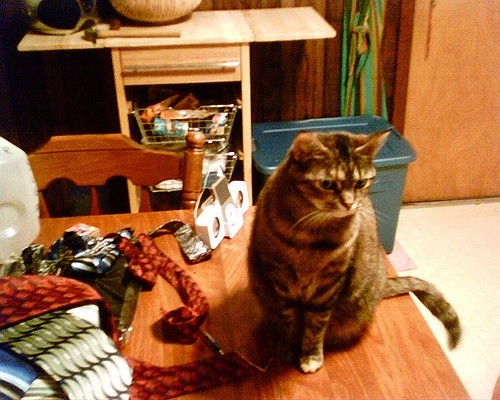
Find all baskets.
<box><xmin>148</xmin><ymin>152</ymin><xmax>238</xmax><ymax>192</ymax></box>
<box><xmin>132</xmin><ymin>103</ymin><xmax>242</xmax><ymax>152</ymax></box>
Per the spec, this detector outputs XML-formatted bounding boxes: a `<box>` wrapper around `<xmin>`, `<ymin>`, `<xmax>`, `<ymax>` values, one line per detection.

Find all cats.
<box><xmin>247</xmin><ymin>127</ymin><xmax>461</xmax><ymax>374</ymax></box>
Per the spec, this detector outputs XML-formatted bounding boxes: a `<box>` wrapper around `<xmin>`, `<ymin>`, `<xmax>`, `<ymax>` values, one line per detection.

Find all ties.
<box><xmin>0</xmin><ymin>219</ymin><xmax>250</xmax><ymax>400</ymax></box>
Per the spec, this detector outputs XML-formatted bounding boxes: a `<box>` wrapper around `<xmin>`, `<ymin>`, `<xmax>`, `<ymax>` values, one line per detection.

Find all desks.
<box><xmin>0</xmin><ymin>199</ymin><xmax>475</xmax><ymax>400</ymax></box>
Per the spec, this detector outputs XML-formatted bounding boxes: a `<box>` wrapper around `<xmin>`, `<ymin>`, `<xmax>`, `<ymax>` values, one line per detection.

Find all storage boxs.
<box><xmin>248</xmin><ymin>111</ymin><xmax>418</xmax><ymax>262</ymax></box>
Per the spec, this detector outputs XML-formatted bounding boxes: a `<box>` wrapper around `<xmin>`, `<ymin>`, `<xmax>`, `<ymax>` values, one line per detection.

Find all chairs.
<box><xmin>22</xmin><ymin>128</ymin><xmax>206</xmax><ymax>219</ymax></box>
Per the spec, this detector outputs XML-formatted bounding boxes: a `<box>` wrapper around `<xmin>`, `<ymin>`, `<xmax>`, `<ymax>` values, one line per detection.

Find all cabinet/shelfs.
<box><xmin>110</xmin><ymin>31</ymin><xmax>257</xmax><ymax>213</ymax></box>
